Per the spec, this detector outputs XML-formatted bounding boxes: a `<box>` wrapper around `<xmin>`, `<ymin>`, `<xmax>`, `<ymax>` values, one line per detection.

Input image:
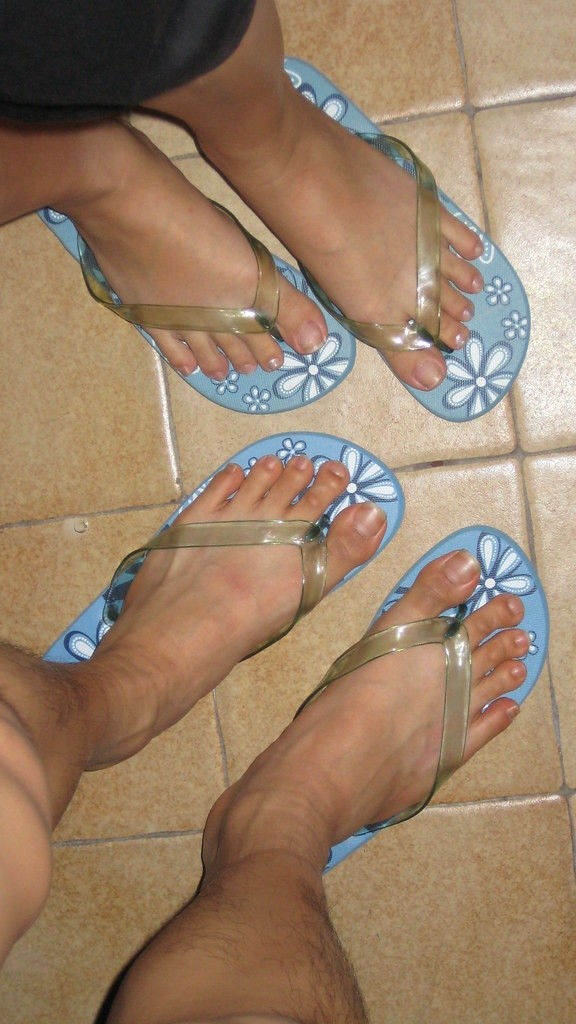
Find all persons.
<box><xmin>0</xmin><ymin>1</ymin><xmax>530</xmax><ymax>422</ymax></box>
<box><xmin>1</xmin><ymin>433</ymin><xmax>550</xmax><ymax>1023</ymax></box>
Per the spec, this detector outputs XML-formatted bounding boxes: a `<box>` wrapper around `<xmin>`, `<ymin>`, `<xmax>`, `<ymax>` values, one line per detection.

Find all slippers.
<box><xmin>290</xmin><ymin>522</ymin><xmax>549</xmax><ymax>878</ymax></box>
<box><xmin>37</xmin><ymin>208</ymin><xmax>355</xmax><ymax>412</ymax></box>
<box><xmin>284</xmin><ymin>52</ymin><xmax>532</xmax><ymax>420</ymax></box>
<box><xmin>41</xmin><ymin>433</ymin><xmax>404</xmax><ymax>665</ymax></box>
